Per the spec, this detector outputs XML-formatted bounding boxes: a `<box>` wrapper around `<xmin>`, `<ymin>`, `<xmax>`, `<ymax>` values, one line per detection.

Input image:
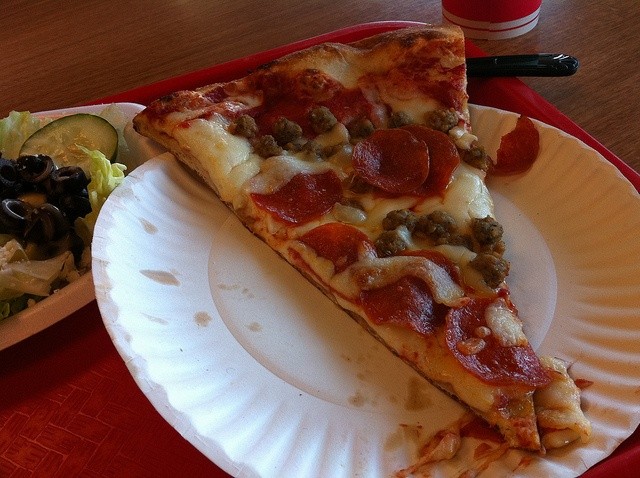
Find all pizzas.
<box><xmin>131</xmin><ymin>24</ymin><xmax>593</xmax><ymax>454</ymax></box>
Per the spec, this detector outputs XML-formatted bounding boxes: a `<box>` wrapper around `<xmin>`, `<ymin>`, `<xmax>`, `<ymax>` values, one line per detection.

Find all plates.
<box><xmin>89</xmin><ymin>102</ymin><xmax>639</xmax><ymax>477</ymax></box>
<box><xmin>2</xmin><ymin>102</ymin><xmax>146</xmax><ymax>349</ymax></box>
